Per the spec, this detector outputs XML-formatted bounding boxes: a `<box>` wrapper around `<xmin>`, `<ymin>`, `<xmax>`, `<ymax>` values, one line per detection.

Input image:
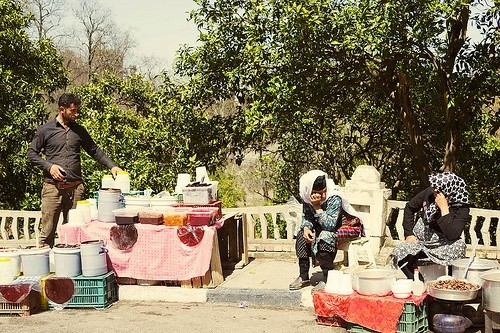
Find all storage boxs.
<box><xmin>317</xmin><ymin>295</ymin><xmax>428</xmax><ymax>333</ymax></box>
<box><xmin>41</xmin><ymin>271</ymin><xmax>120</xmax><ymax>311</ymax></box>
<box><xmin>0</xmin><ymin>277</ymin><xmax>39</xmax><ymax>317</ymax></box>
<box><xmin>112</xmin><ymin>181</ymin><xmax>222</xmax><ymax>227</ymax></box>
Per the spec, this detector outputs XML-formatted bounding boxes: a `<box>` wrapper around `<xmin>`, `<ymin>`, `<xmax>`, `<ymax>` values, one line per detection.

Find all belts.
<box><xmin>44</xmin><ymin>177</ymin><xmax>83</xmax><ymax>191</ymax></box>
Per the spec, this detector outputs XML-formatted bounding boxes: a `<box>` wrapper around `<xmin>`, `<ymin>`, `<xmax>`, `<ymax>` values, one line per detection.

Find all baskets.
<box><xmin>345</xmin><ymin>296</ymin><xmax>429</xmax><ymax>333</ymax></box>
<box><xmin>0</xmin><ymin>277</ymin><xmax>49</xmax><ymax>318</ymax></box>
<box><xmin>41</xmin><ymin>272</ymin><xmax>119</xmax><ymax>309</ymax></box>
<box><xmin>310</xmin><ymin>291</ymin><xmax>347</xmax><ymax>328</ymax></box>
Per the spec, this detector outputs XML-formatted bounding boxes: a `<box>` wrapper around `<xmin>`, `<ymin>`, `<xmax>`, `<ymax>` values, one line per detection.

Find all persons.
<box><xmin>390</xmin><ymin>171</ymin><xmax>470</xmax><ymax>282</ymax></box>
<box><xmin>289</xmin><ymin>170</ymin><xmax>366</xmax><ymax>290</ymax></box>
<box><xmin>28</xmin><ymin>93</ymin><xmax>123</xmax><ymax>250</ymax></box>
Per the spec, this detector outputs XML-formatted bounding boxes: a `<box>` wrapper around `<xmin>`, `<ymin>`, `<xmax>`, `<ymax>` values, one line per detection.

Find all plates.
<box><xmin>427</xmin><ymin>279</ymin><xmax>480</xmax><ymax>301</ymax></box>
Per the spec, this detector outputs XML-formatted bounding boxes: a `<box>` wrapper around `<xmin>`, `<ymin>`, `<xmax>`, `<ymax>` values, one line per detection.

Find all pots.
<box><xmin>477</xmin><ymin>272</ymin><xmax>500</xmax><ymax>312</ymax></box>
<box><xmin>450</xmin><ymin>259</ymin><xmax>497</xmax><ymax>285</ymax></box>
<box><xmin>355</xmin><ymin>270</ymin><xmax>394</xmax><ymax>296</ymax></box>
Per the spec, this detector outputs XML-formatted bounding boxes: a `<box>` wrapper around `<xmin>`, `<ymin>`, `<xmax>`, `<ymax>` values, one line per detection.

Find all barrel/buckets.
<box><xmin>53</xmin><ymin>244</ymin><xmax>81</xmax><ymax>277</ymax></box>
<box><xmin>0</xmin><ymin>248</ymin><xmax>50</xmax><ymax>277</ymax></box>
<box><xmin>81</xmin><ymin>240</ymin><xmax>108</xmax><ymax>277</ymax></box>
<box><xmin>97</xmin><ymin>190</ymin><xmax>122</xmax><ymax>222</ymax></box>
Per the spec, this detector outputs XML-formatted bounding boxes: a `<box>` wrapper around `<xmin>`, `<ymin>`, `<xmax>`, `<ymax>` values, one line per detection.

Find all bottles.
<box><xmin>76</xmin><ymin>201</ymin><xmax>91</xmax><ymax>223</ymax></box>
<box><xmin>102</xmin><ymin>172</ymin><xmax>130</xmax><ymax>193</ymax></box>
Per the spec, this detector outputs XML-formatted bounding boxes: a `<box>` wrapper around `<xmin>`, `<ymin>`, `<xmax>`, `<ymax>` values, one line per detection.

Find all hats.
<box><xmin>312</xmin><ymin>175</ymin><xmax>326</xmax><ymax>191</ymax></box>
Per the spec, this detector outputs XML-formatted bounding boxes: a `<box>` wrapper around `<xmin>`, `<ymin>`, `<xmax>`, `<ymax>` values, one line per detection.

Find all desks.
<box><xmin>57</xmin><ymin>211</ymin><xmax>249</xmax><ymax>289</ymax></box>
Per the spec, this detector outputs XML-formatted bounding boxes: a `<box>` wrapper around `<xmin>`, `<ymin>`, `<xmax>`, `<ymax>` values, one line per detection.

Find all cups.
<box><xmin>324</xmin><ymin>270</ymin><xmax>353</xmax><ymax>296</ymax></box>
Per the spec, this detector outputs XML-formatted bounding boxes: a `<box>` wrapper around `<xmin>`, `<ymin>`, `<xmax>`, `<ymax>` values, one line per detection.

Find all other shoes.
<box><xmin>289</xmin><ymin>275</ymin><xmax>312</xmax><ymax>290</ymax></box>
<box><xmin>311</xmin><ymin>280</ymin><xmax>326</xmax><ymax>295</ymax></box>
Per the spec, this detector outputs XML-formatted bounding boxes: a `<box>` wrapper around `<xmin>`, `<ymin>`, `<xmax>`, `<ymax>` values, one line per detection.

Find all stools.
<box><xmin>343</xmin><ymin>237</ymin><xmax>376</xmax><ymax>268</ymax></box>
<box><xmin>414</xmin><ymin>257</ymin><xmax>448</xmax><ymax>280</ymax></box>
